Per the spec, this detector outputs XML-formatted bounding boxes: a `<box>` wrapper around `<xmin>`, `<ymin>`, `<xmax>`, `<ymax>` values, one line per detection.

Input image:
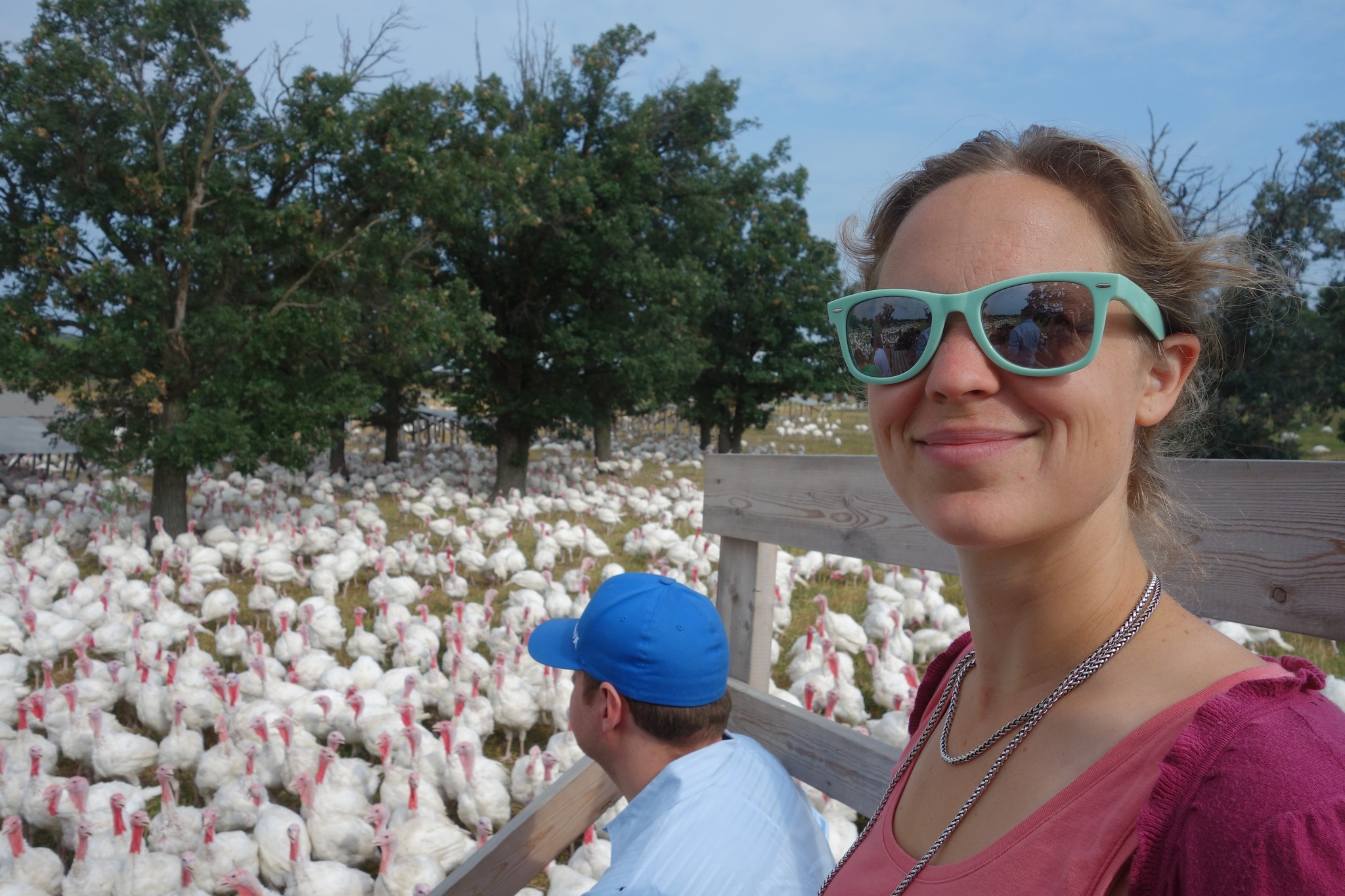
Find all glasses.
<box><xmin>827</xmin><ymin>271</ymin><xmax>1167</xmax><ymax>386</ymax></box>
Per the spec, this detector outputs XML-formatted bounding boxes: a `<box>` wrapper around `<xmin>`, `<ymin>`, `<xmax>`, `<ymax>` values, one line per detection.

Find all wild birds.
<box><xmin>16</xmin><ymin>416</ymin><xmax>1345</xmax><ymax>896</ymax></box>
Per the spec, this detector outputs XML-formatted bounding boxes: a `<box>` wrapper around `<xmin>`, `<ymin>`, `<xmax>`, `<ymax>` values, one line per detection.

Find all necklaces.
<box><xmin>813</xmin><ymin>571</ymin><xmax>1164</xmax><ymax>895</ymax></box>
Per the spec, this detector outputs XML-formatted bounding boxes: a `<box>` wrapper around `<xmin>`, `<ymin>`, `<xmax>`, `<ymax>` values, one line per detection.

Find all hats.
<box><xmin>527</xmin><ymin>571</ymin><xmax>730</xmax><ymax>708</ymax></box>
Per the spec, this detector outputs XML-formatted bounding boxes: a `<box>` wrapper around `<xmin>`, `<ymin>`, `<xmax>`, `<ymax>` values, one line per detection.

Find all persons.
<box><xmin>815</xmin><ymin>122</ymin><xmax>1344</xmax><ymax>895</ymax></box>
<box><xmin>525</xmin><ymin>571</ymin><xmax>840</xmax><ymax>896</ymax></box>
<box><xmin>854</xmin><ymin>304</ymin><xmax>1085</xmax><ymax>379</ymax></box>
